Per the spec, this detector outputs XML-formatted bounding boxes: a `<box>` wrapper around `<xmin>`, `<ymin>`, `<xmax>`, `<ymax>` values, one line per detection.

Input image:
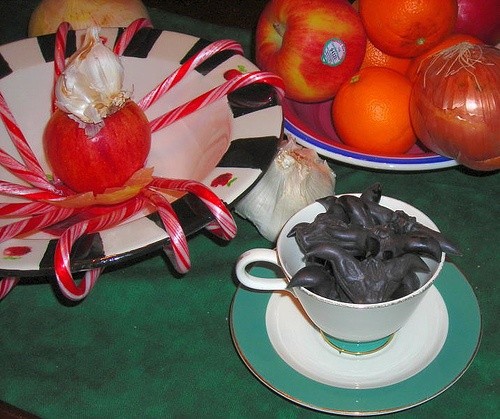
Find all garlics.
<box><xmin>55</xmin><ymin>25</ymin><xmax>134</xmax><ymax>137</ymax></box>
<box><xmin>229</xmin><ymin>136</ymin><xmax>336</xmax><ymax>243</ymax></box>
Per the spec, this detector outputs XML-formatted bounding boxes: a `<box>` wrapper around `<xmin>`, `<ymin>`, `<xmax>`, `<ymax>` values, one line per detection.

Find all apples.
<box><xmin>457</xmin><ymin>1</ymin><xmax>500</xmax><ymax>45</ymax></box>
<box><xmin>43</xmin><ymin>98</ymin><xmax>152</xmax><ymax>196</ymax></box>
<box><xmin>256</xmin><ymin>0</ymin><xmax>368</xmax><ymax>102</ymax></box>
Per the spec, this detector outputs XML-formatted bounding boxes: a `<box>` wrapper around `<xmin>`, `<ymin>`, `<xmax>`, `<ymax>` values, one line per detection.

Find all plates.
<box><xmin>282</xmin><ymin>86</ymin><xmax>461</xmax><ymax>171</ymax></box>
<box><xmin>229</xmin><ymin>244</ymin><xmax>484</xmax><ymax>417</ymax></box>
<box><xmin>1</xmin><ymin>27</ymin><xmax>285</xmax><ymax>275</ymax></box>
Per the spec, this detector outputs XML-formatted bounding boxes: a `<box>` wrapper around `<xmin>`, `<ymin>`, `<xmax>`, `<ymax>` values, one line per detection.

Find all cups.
<box><xmin>235</xmin><ymin>192</ymin><xmax>446</xmax><ymax>356</ymax></box>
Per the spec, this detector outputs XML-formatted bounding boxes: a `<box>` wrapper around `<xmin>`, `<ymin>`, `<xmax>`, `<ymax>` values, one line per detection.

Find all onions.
<box><xmin>408</xmin><ymin>41</ymin><xmax>500</xmax><ymax>170</ymax></box>
<box><xmin>28</xmin><ymin>0</ymin><xmax>155</xmax><ymax>39</ymax></box>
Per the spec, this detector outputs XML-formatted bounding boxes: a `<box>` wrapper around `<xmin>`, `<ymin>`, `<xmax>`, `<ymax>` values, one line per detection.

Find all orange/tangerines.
<box><xmin>332</xmin><ymin>1</ymin><xmax>483</xmax><ymax>155</ymax></box>
